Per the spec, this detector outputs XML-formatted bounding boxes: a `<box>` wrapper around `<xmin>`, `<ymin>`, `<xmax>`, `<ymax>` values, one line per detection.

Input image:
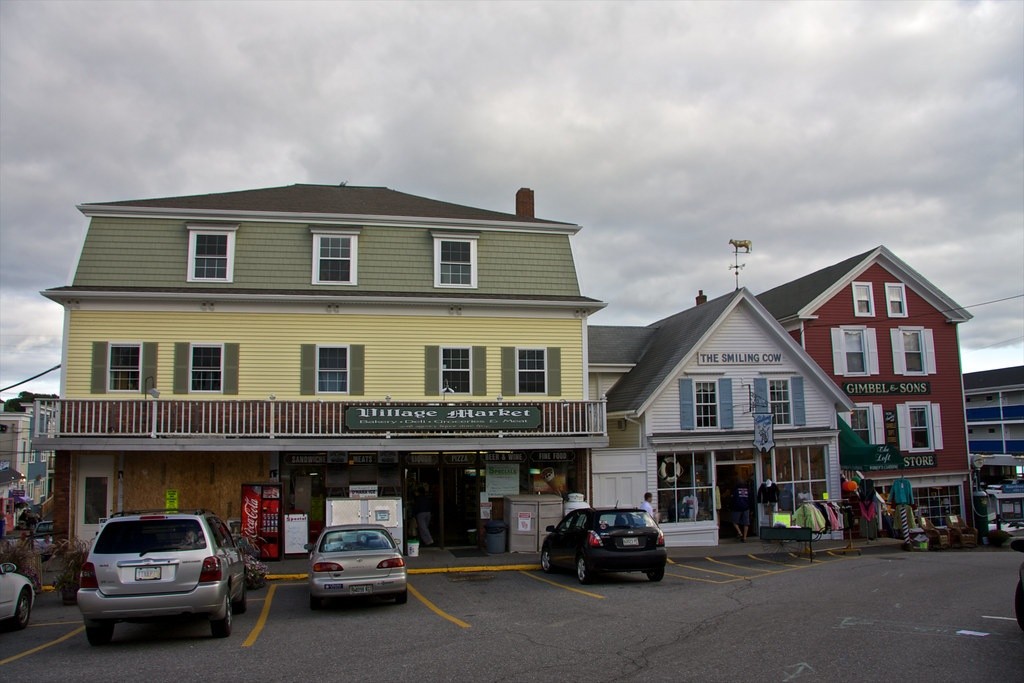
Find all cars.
<box><xmin>303</xmin><ymin>524</ymin><xmax>408</xmax><ymax>610</ymax></box>
<box><xmin>540</xmin><ymin>507</ymin><xmax>667</xmax><ymax>585</ymax></box>
<box><xmin>0</xmin><ymin>563</ymin><xmax>35</xmax><ymax>630</ymax></box>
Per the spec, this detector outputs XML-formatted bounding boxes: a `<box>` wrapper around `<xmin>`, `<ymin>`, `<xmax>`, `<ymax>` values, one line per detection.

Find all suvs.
<box><xmin>76</xmin><ymin>508</ymin><xmax>248</xmax><ymax>646</ymax></box>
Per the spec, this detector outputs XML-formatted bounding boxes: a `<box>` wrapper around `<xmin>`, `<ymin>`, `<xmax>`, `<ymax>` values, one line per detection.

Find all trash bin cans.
<box><xmin>485</xmin><ymin>520</ymin><xmax>506</xmax><ymax>553</ymax></box>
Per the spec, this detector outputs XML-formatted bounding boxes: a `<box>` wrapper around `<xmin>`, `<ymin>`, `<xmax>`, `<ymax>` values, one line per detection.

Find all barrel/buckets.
<box><xmin>407</xmin><ymin>540</ymin><xmax>419</xmax><ymax>556</ymax></box>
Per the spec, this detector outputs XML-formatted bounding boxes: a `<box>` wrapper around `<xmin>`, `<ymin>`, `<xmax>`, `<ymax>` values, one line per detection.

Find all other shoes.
<box><xmin>426</xmin><ymin>540</ymin><xmax>435</xmax><ymax>547</ymax></box>
<box><xmin>737</xmin><ymin>533</ymin><xmax>743</xmax><ymax>539</ymax></box>
<box><xmin>741</xmin><ymin>538</ymin><xmax>747</xmax><ymax>543</ymax></box>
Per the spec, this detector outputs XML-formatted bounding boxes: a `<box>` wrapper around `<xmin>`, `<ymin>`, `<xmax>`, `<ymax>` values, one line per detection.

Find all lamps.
<box><xmin>144</xmin><ymin>376</ymin><xmax>159</xmax><ymax>400</ymax></box>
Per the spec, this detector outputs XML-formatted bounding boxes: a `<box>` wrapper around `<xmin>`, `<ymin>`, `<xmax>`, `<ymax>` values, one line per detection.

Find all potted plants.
<box><xmin>51</xmin><ymin>573</ymin><xmax>79</xmax><ymax>606</ymax></box>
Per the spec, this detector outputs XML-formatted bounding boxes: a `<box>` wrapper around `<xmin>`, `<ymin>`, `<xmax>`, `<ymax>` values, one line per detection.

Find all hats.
<box><xmin>736</xmin><ymin>479</ymin><xmax>747</xmax><ymax>488</ymax></box>
<box><xmin>23</xmin><ymin>508</ymin><xmax>27</xmax><ymax>510</ymax></box>
<box><xmin>27</xmin><ymin>508</ymin><xmax>32</xmax><ymax>511</ymax></box>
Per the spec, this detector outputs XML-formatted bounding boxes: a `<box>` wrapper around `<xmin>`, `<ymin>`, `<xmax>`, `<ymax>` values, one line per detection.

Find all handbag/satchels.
<box><xmin>408</xmin><ymin>518</ymin><xmax>417</xmax><ymax>537</ymax></box>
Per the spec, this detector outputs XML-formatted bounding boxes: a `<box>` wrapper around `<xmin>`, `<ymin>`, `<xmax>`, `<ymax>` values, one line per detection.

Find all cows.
<box><xmin>728</xmin><ymin>239</ymin><xmax>752</xmax><ymax>253</ymax></box>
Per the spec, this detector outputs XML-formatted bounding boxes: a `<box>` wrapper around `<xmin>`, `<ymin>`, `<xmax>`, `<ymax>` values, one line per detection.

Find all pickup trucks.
<box><xmin>7</xmin><ymin>521</ymin><xmax>53</xmax><ymax>554</ymax></box>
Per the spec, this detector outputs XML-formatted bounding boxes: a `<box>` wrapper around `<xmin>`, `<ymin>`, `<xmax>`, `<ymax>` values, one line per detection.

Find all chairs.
<box><xmin>945</xmin><ymin>514</ymin><xmax>977</xmax><ymax>550</ymax></box>
<box><xmin>916</xmin><ymin>516</ymin><xmax>950</xmax><ymax>552</ymax></box>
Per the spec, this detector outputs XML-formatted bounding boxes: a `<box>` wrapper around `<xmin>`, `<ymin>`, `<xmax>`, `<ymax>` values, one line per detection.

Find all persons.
<box><xmin>757</xmin><ymin>479</ymin><xmax>779</xmax><ymax>504</ymax></box>
<box><xmin>728</xmin><ymin>474</ymin><xmax>751</xmax><ymax>543</ymax></box>
<box><xmin>640</xmin><ymin>492</ymin><xmax>657</xmax><ymax>518</ymax></box>
<box><xmin>17</xmin><ymin>508</ymin><xmax>42</xmax><ymax>530</ymax></box>
<box><xmin>415</xmin><ymin>494</ymin><xmax>434</xmax><ymax>545</ymax></box>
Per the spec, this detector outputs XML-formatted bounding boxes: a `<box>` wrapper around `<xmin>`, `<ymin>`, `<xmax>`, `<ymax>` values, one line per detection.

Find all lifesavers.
<box><xmin>660</xmin><ymin>457</ymin><xmax>681</xmax><ymax>483</ymax></box>
